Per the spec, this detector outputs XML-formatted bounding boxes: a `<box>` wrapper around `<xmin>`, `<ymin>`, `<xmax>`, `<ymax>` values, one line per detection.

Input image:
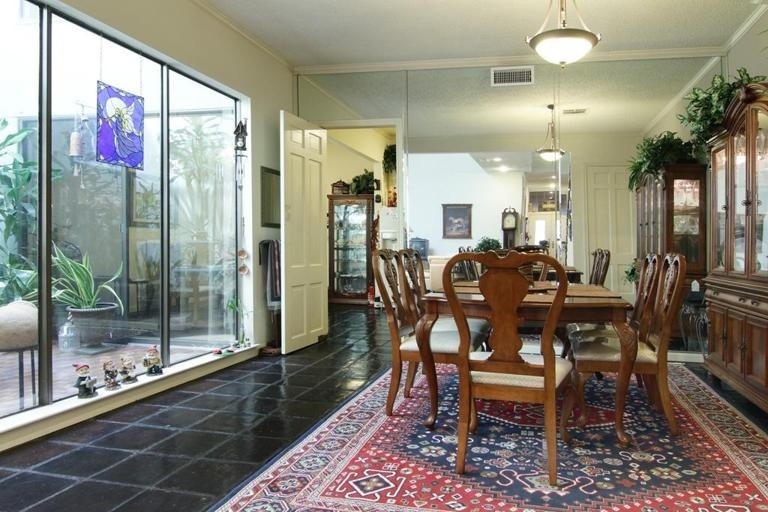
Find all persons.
<box><xmin>141</xmin><ymin>345</ymin><xmax>163</xmax><ymax>377</ymax></box>
<box><xmin>70</xmin><ymin>361</ymin><xmax>98</xmax><ymax>399</ymax></box>
<box><xmin>119</xmin><ymin>357</ymin><xmax>138</xmax><ymax>385</ymax></box>
<box><xmin>101</xmin><ymin>360</ymin><xmax>121</xmax><ymax>391</ymax></box>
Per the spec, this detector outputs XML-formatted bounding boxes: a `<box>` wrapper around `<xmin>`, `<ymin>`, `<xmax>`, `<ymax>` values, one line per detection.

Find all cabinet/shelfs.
<box><xmin>328</xmin><ymin>192</ymin><xmax>373</xmax><ymax>307</ymax></box>
<box><xmin>636</xmin><ymin>167</ymin><xmax>706</xmax><ymax>296</ymax></box>
<box><xmin>700</xmin><ymin>82</ymin><xmax>768</xmax><ymax>420</ymax></box>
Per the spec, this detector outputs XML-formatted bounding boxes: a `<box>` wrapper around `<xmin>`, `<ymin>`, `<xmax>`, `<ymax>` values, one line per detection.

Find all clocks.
<box><xmin>502</xmin><ymin>205</ymin><xmax>518</xmax><ymax>230</ymax></box>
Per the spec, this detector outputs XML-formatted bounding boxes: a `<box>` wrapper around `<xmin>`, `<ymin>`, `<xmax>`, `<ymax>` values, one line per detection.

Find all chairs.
<box><xmin>0</xmin><ymin>297</ymin><xmax>42</xmax><ymax>399</ymax></box>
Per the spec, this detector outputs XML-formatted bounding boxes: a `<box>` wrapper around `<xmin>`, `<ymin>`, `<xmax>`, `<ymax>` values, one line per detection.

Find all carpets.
<box><xmin>205</xmin><ymin>351</ymin><xmax>768</xmax><ymax>511</ymax></box>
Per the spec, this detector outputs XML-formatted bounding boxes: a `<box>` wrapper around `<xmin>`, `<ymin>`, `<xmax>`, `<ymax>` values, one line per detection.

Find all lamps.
<box><xmin>233</xmin><ymin>120</ymin><xmax>249</xmax><ymax>158</ymax></box>
<box><xmin>65</xmin><ymin>102</ymin><xmax>114</xmax><ymax>185</ymax></box>
<box><xmin>536</xmin><ymin>102</ymin><xmax>566</xmax><ymax>164</ymax></box>
<box><xmin>522</xmin><ymin>3</ymin><xmax>600</xmax><ymax>70</ymax></box>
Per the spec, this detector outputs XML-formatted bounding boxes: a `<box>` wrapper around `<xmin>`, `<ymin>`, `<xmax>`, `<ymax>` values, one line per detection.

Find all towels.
<box><xmin>258</xmin><ymin>239</ymin><xmax>281</xmax><ymax>316</ymax></box>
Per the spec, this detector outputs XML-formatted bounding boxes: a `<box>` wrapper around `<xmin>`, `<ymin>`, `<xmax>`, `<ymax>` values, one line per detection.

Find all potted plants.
<box><xmin>22</xmin><ymin>241</ymin><xmax>125</xmax><ymax>347</ymax></box>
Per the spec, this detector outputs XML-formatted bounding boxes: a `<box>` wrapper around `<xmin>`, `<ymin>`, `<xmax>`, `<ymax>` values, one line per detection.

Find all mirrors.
<box><xmin>260</xmin><ymin>164</ymin><xmax>281</xmax><ymax>227</ymax></box>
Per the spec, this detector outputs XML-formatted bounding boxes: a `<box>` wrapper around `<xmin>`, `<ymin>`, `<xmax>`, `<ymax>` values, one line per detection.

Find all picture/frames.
<box><xmin>441</xmin><ymin>201</ymin><xmax>472</xmax><ymax>239</ymax></box>
